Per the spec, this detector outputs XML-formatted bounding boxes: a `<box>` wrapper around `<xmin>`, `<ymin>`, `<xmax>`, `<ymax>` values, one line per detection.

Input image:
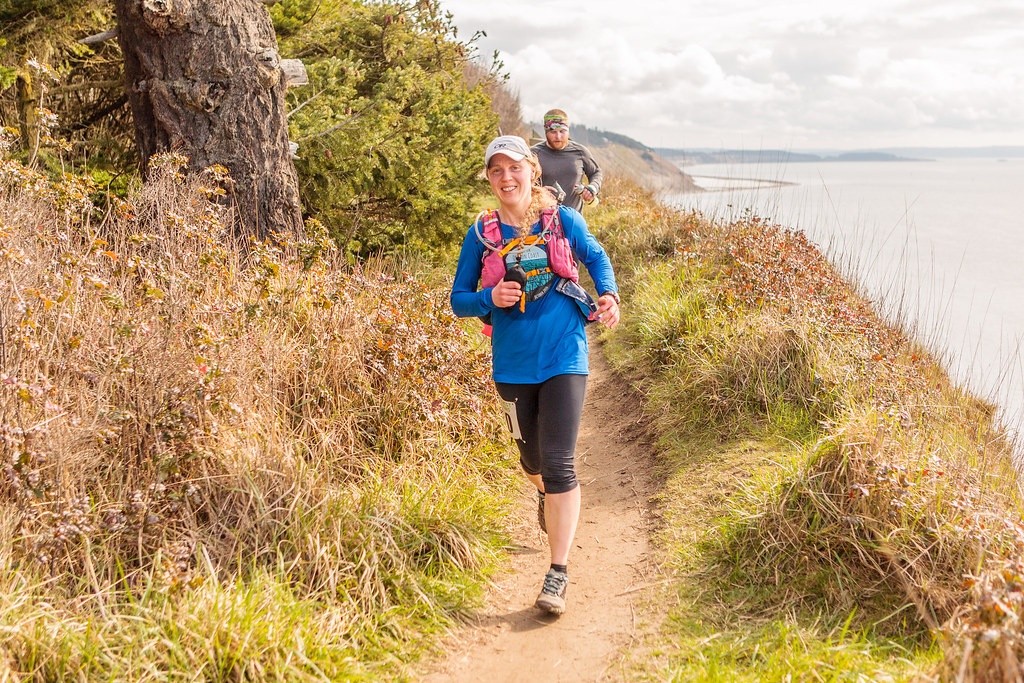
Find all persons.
<box><xmin>448</xmin><ymin>132</ymin><xmax>620</xmax><ymax>613</ymax></box>
<box><xmin>529</xmin><ymin>108</ymin><xmax>603</xmax><ymax>269</ymax></box>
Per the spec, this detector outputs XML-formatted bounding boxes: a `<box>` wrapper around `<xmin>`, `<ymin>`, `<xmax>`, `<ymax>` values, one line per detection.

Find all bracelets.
<box><xmin>602</xmin><ymin>291</ymin><xmax>620</xmax><ymax>304</ymax></box>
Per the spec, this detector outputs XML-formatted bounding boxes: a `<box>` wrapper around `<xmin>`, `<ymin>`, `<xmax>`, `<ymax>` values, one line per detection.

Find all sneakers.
<box><xmin>537</xmin><ymin>489</ymin><xmax>548</xmax><ymax>535</ymax></box>
<box><xmin>533</xmin><ymin>568</ymin><xmax>569</xmax><ymax>614</ymax></box>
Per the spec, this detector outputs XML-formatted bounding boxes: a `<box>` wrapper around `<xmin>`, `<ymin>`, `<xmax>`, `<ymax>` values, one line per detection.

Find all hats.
<box><xmin>484</xmin><ymin>135</ymin><xmax>531</xmax><ymax>165</ymax></box>
